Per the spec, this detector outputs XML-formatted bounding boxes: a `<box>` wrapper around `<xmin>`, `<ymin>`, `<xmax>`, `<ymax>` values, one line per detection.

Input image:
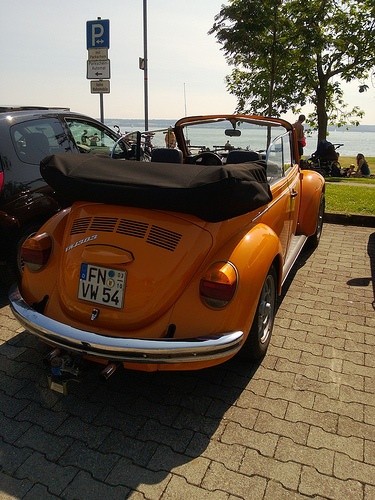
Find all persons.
<box><xmin>290</xmin><ymin>114</ymin><xmax>306</xmax><ymax>164</ymax></box>
<box><xmin>351</xmin><ymin>152</ymin><xmax>370</xmax><ymax>178</ymax></box>
<box><xmin>225</xmin><ymin>140</ymin><xmax>231</xmax><ymax>146</ymax></box>
<box><xmin>81</xmin><ymin>130</ymin><xmax>101</xmax><ymax>146</ymax></box>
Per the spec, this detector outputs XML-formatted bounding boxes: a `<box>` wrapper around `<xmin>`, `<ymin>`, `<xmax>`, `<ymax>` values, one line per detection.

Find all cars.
<box><xmin>5</xmin><ymin>112</ymin><xmax>327</xmax><ymax>394</ymax></box>
<box><xmin>0</xmin><ymin>106</ymin><xmax>131</xmax><ymax>302</ymax></box>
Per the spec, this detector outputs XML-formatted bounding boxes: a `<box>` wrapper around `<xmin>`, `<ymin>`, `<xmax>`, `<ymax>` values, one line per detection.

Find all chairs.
<box><xmin>20</xmin><ymin>133</ymin><xmax>50</xmax><ymax>160</ymax></box>
<box><xmin>226</xmin><ymin>151</ymin><xmax>262</xmax><ymax>164</ymax></box>
<box><xmin>149</xmin><ymin>148</ymin><xmax>183</xmax><ymax>163</ymax></box>
